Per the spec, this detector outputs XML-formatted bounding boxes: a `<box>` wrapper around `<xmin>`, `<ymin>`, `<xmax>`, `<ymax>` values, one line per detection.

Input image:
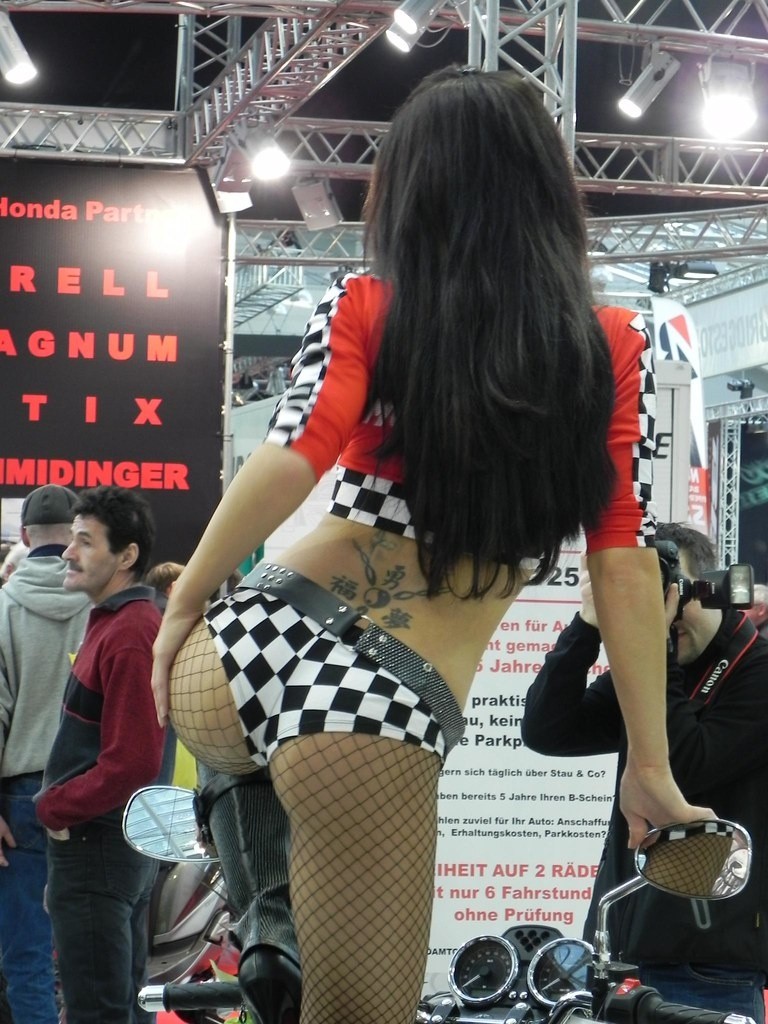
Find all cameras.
<box><xmin>642</xmin><ymin>536</ymin><xmax>754</xmax><ymax>622</ymax></box>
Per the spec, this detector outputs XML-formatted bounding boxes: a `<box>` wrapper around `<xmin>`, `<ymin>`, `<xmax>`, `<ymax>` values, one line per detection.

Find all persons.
<box><xmin>522</xmin><ymin>522</ymin><xmax>767</xmax><ymax>1024</ymax></box>
<box><xmin>151</xmin><ymin>69</ymin><xmax>721</xmax><ymax>1024</ymax></box>
<box><xmin>1</xmin><ymin>484</ymin><xmax>186</xmax><ymax>1024</ymax></box>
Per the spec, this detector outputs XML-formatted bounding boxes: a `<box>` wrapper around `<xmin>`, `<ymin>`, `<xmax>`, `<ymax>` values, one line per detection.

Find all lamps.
<box><xmin>696</xmin><ymin>60</ymin><xmax>759</xmax><ymax>140</ymax></box>
<box><xmin>386</xmin><ymin>12</ymin><xmax>440</xmax><ymax>53</ymax></box>
<box><xmin>648</xmin><ymin>263</ymin><xmax>665</xmax><ymax>294</ymax></box>
<box><xmin>673</xmin><ymin>262</ymin><xmax>719</xmax><ymax>276</ymax></box>
<box><xmin>0</xmin><ymin>11</ymin><xmax>37</xmax><ymax>84</ymax></box>
<box><xmin>207</xmin><ymin>165</ymin><xmax>253</xmax><ymax>214</ymax></box>
<box><xmin>291</xmin><ymin>178</ymin><xmax>344</xmax><ymax>231</ymax></box>
<box><xmin>726</xmin><ymin>379</ymin><xmax>755</xmax><ymax>400</ymax></box>
<box><xmin>229</xmin><ymin>130</ymin><xmax>290</xmax><ymax>180</ymax></box>
<box><xmin>617</xmin><ymin>51</ymin><xmax>681</xmax><ymax>119</ymax></box>
<box><xmin>393</xmin><ymin>0</ymin><xmax>438</xmax><ymax>35</ymax></box>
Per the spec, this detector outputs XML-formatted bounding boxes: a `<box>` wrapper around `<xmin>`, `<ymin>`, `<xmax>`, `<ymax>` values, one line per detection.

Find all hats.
<box><xmin>21</xmin><ymin>484</ymin><xmax>82</xmax><ymax>526</ymax></box>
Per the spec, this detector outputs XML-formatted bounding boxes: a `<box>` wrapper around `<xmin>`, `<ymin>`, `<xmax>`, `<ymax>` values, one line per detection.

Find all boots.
<box><xmin>192</xmin><ymin>762</ymin><xmax>303</xmax><ymax>1024</ymax></box>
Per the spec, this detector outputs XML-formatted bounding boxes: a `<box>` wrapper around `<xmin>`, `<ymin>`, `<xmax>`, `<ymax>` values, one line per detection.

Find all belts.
<box><xmin>235</xmin><ymin>562</ymin><xmax>465</xmax><ymax>755</ymax></box>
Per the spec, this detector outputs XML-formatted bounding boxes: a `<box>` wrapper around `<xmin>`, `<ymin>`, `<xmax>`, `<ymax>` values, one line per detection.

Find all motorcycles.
<box><xmin>123</xmin><ymin>783</ymin><xmax>754</xmax><ymax>1022</ymax></box>
